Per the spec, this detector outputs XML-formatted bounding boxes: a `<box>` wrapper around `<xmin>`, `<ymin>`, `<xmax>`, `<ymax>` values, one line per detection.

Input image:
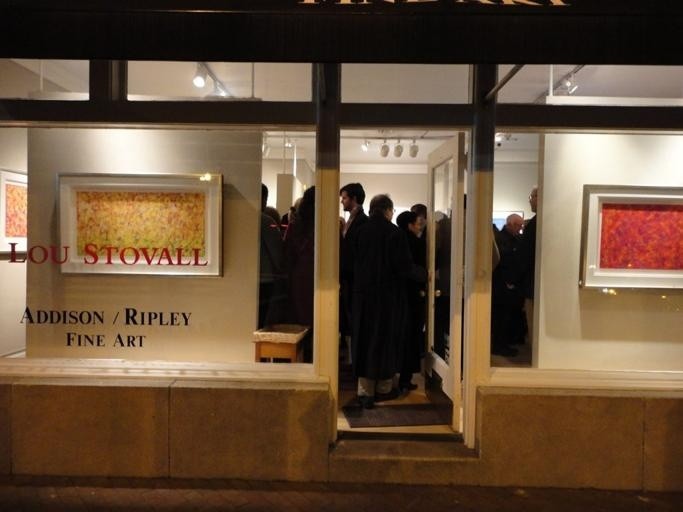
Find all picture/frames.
<box><xmin>578</xmin><ymin>183</ymin><xmax>683</xmax><ymax>291</ymax></box>
<box><xmin>55</xmin><ymin>171</ymin><xmax>224</xmax><ymax>279</ymax></box>
<box><xmin>0</xmin><ymin>168</ymin><xmax>28</xmax><ymax>254</ymax></box>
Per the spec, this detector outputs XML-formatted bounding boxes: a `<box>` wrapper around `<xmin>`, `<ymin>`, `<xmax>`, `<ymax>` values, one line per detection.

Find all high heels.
<box><xmin>398</xmin><ymin>377</ymin><xmax>418</xmax><ymax>394</ymax></box>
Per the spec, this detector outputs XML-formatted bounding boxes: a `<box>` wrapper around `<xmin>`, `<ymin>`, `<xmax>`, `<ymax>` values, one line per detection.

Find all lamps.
<box><xmin>283</xmin><ymin>135</ymin><xmax>296</xmax><ymax>149</ymax></box>
<box><xmin>193</xmin><ymin>62</ymin><xmax>234</xmax><ymax>98</ymax></box>
<box><xmin>360</xmin><ymin>137</ymin><xmax>419</xmax><ymax>157</ymax></box>
<box><xmin>494</xmin><ymin>132</ymin><xmax>505</xmax><ymax>142</ymax></box>
<box><xmin>560</xmin><ymin>77</ymin><xmax>579</xmax><ymax>96</ymax></box>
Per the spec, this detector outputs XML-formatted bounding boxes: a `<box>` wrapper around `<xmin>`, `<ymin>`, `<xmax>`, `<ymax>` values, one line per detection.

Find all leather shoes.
<box><xmin>357</xmin><ymin>396</ymin><xmax>374</xmax><ymax>409</ymax></box>
<box><xmin>373</xmin><ymin>387</ymin><xmax>399</xmax><ymax>402</ymax></box>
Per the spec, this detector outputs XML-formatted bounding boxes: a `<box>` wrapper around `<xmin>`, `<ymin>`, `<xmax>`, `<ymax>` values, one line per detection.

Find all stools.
<box><xmin>253</xmin><ymin>323</ymin><xmax>309</xmax><ymax>362</ymax></box>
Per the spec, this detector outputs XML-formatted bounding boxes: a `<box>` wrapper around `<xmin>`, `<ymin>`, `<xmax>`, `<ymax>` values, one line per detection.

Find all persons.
<box><xmin>257</xmin><ymin>182</ymin><xmax>539</xmax><ymax>413</ymax></box>
